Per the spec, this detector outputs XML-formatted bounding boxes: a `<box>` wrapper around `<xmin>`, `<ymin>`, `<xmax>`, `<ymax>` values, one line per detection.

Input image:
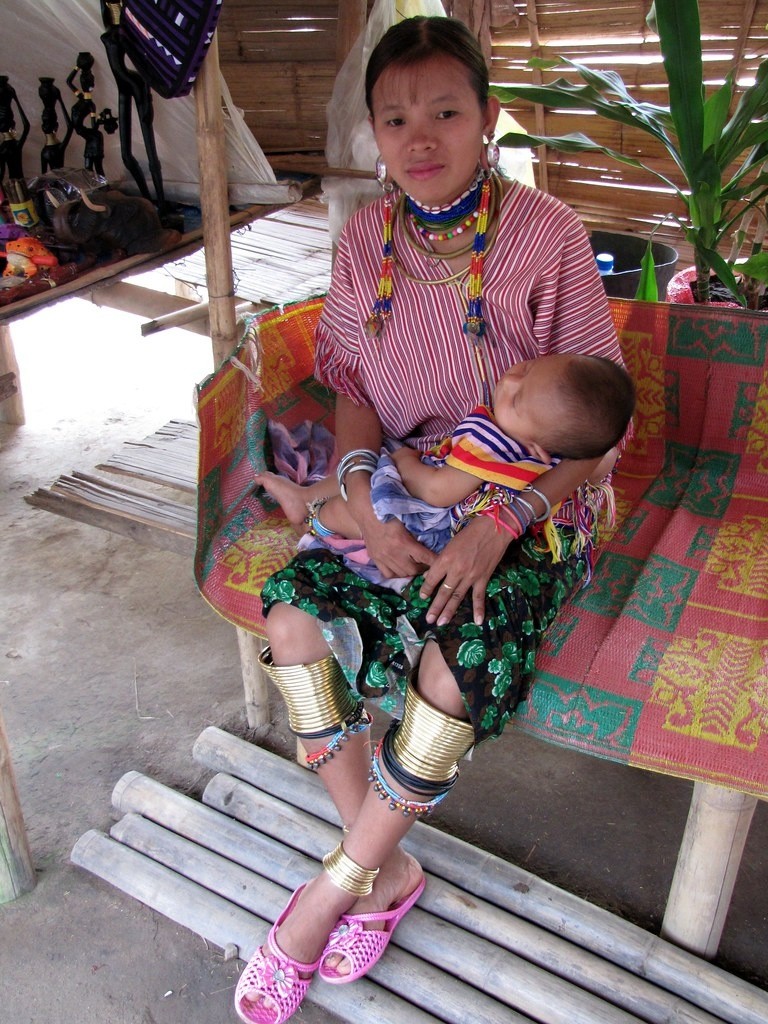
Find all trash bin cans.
<box><xmin>588</xmin><ymin>230</ymin><xmax>679</xmax><ymax>303</ymax></box>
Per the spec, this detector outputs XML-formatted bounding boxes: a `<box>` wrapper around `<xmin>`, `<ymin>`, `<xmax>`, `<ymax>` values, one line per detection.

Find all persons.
<box><xmin>230</xmin><ymin>13</ymin><xmax>632</xmax><ymax>1024</ymax></box>
<box><xmin>252</xmin><ymin>354</ymin><xmax>633</xmax><ymax>566</ymax></box>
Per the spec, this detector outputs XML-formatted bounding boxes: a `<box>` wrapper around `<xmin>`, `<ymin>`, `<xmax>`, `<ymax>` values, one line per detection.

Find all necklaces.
<box><xmin>361</xmin><ymin>164</ymin><xmax>500</xmax><ymax>414</ymax></box>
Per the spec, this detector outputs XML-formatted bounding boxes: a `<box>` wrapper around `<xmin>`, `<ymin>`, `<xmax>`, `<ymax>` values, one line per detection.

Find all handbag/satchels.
<box><xmin>119</xmin><ymin>0</ymin><xmax>223</xmax><ymax>99</ymax></box>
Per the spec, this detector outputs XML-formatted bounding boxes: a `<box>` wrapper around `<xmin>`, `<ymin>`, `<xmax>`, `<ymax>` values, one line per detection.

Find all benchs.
<box><xmin>193</xmin><ymin>293</ymin><xmax>768</xmax><ymax>961</ymax></box>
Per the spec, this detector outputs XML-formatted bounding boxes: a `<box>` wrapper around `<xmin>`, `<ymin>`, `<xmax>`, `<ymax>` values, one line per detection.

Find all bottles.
<box><xmin>596</xmin><ymin>253</ymin><xmax>615</xmax><ymax>275</ymax></box>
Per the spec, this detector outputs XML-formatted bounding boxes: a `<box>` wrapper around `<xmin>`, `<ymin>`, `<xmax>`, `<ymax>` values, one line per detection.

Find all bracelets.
<box><xmin>338</xmin><ymin>448</ymin><xmax>380</xmax><ymax>499</ymax></box>
<box><xmin>482</xmin><ymin>487</ymin><xmax>551</xmax><ymax>539</ymax></box>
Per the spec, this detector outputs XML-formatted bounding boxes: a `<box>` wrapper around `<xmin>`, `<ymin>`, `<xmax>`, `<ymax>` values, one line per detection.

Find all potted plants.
<box><xmin>486</xmin><ymin>0</ymin><xmax>768</xmax><ymax>311</ymax></box>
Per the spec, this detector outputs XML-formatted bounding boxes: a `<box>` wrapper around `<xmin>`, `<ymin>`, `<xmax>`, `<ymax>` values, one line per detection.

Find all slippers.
<box><xmin>234</xmin><ymin>882</ymin><xmax>320</xmax><ymax>1024</ymax></box>
<box><xmin>318</xmin><ymin>875</ymin><xmax>426</xmax><ymax>984</ymax></box>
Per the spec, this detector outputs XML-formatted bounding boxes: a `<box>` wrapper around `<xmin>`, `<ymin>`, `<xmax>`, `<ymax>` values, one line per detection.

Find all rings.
<box><xmin>441</xmin><ymin>581</ymin><xmax>454</xmax><ymax>592</ymax></box>
